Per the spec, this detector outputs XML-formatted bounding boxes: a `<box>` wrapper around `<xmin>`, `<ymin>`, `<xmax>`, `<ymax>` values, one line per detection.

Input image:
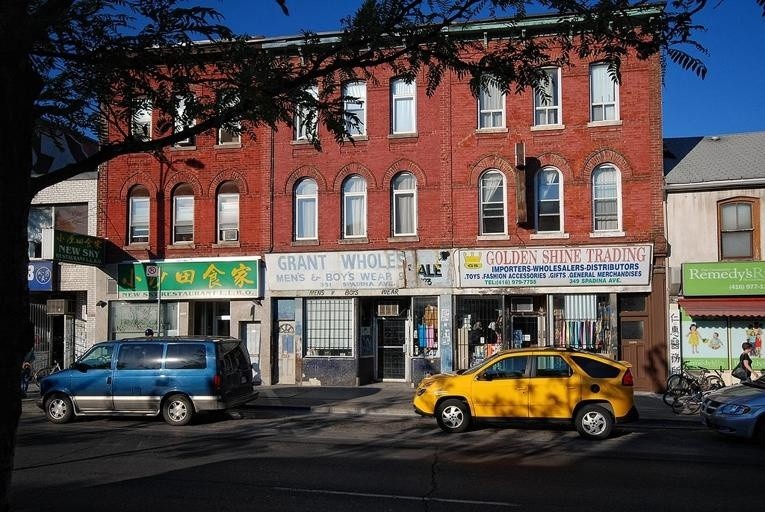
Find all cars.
<box><xmin>700</xmin><ymin>368</ymin><xmax>765</xmax><ymax>442</ymax></box>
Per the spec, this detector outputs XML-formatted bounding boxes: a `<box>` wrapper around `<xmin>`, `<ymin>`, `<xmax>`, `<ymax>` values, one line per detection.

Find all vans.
<box><xmin>37</xmin><ymin>335</ymin><xmax>259</xmax><ymax>426</ymax></box>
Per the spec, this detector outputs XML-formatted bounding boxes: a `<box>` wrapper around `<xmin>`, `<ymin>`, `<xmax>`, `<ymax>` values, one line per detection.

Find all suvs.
<box><xmin>413</xmin><ymin>348</ymin><xmax>633</xmax><ymax>441</ymax></box>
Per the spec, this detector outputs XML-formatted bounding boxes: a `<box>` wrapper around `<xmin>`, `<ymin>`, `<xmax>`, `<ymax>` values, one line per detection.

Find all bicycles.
<box><xmin>33</xmin><ymin>354</ymin><xmax>79</xmax><ymax>389</ymax></box>
<box><xmin>663</xmin><ymin>361</ymin><xmax>725</xmax><ymax>415</ymax></box>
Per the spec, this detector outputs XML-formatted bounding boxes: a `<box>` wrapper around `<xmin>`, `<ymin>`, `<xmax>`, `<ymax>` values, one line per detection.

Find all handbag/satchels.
<box><xmin>731</xmin><ymin>363</ymin><xmax>747</xmax><ymax>380</ymax></box>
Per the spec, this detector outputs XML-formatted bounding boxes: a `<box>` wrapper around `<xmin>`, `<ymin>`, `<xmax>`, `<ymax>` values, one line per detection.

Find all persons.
<box><xmin>740</xmin><ymin>342</ymin><xmax>759</xmax><ymax>385</ymax></box>
<box><xmin>21</xmin><ymin>345</ymin><xmax>36</xmax><ymax>401</ymax></box>
<box><xmin>144</xmin><ymin>328</ymin><xmax>154</xmax><ymax>337</ymax></box>
<box><xmin>686</xmin><ymin>322</ymin><xmax>762</xmax><ymax>357</ymax></box>
